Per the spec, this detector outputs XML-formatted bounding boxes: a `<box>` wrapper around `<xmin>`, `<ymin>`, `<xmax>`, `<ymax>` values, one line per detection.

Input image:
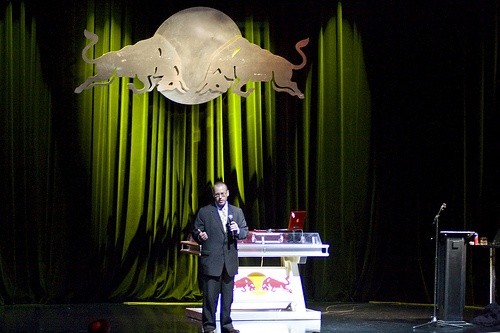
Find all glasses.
<box><xmin>212</xmin><ymin>191</ymin><xmax>227</xmax><ymax>197</ymax></box>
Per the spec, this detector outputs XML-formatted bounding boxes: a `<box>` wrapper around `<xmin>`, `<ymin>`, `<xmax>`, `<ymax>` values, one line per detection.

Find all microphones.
<box><xmin>228</xmin><ymin>214</ymin><xmax>234</xmax><ymax>237</ymax></box>
<box><xmin>432</xmin><ymin>203</ymin><xmax>447</xmax><ymax>225</ymax></box>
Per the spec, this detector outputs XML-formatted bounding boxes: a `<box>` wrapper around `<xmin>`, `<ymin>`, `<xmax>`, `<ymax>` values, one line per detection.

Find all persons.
<box><xmin>192</xmin><ymin>181</ymin><xmax>249</xmax><ymax>333</ymax></box>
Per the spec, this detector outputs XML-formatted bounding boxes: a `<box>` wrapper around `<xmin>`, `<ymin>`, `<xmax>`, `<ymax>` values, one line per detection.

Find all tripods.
<box><xmin>413</xmin><ymin>219</ymin><xmax>463</xmax><ymax>329</ymax></box>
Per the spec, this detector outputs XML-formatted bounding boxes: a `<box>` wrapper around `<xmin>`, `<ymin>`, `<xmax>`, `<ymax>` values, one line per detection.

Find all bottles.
<box><xmin>474</xmin><ymin>233</ymin><xmax>478</xmax><ymax>245</ymax></box>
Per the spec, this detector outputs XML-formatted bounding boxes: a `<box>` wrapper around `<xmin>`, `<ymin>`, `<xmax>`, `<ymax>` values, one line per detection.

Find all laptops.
<box><xmin>269</xmin><ymin>211</ymin><xmax>307</xmax><ymax>231</ymax></box>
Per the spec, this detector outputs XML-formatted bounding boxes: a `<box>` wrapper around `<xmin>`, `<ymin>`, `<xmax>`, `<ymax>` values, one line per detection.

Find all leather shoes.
<box><xmin>221</xmin><ymin>327</ymin><xmax>240</xmax><ymax>333</ymax></box>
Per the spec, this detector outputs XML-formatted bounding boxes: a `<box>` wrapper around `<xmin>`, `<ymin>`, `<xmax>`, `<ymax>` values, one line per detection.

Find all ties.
<box><xmin>221</xmin><ymin>208</ymin><xmax>226</xmax><ymax>230</ymax></box>
